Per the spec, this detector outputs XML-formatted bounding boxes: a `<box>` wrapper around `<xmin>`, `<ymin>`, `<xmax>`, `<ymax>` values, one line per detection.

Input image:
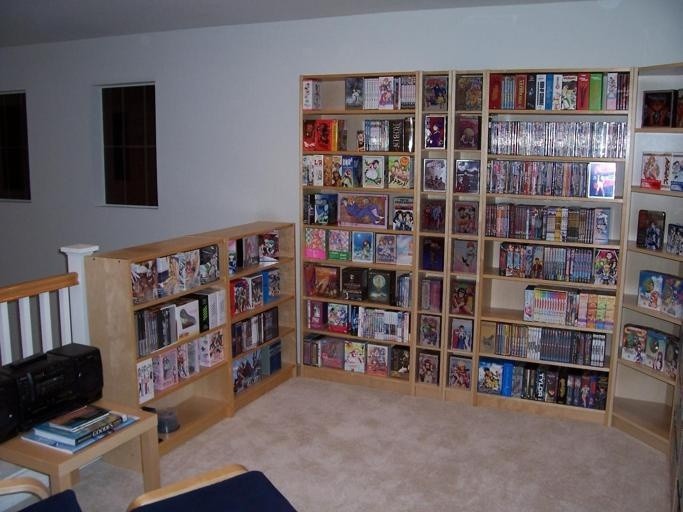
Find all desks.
<box><xmin>8</xmin><ymin>398</ymin><xmax>159</xmax><ymax>497</ymax></box>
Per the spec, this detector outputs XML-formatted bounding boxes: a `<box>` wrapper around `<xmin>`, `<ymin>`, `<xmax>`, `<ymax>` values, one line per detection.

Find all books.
<box><xmin>416</xmin><ymin>75</ymin><xmax>449</xmax><ymax>385</ymax></box>
<box><xmin>33</xmin><ymin>408</ymin><xmax>128</xmax><ymax>447</ymax></box>
<box><xmin>488</xmin><ymin>72</ymin><xmax>630</xmax><ymax>158</ymax></box>
<box><xmin>639</xmin><ymin>152</ymin><xmax>672</xmax><ymax>192</ymax></box>
<box><xmin>500</xmin><ymin>240</ymin><xmax>620</xmax><ymax>330</ymax></box>
<box><xmin>302</xmin><ymin>76</ymin><xmax>414</xmax><ymax>152</ymax></box>
<box><xmin>304</xmin><ymin>300</ymin><xmax>411</xmax><ymax>377</ymax></box>
<box><xmin>304</xmin><ymin>226</ymin><xmax>413</xmax><ymax>308</ymax></box>
<box><xmin>487</xmin><ymin>161</ymin><xmax>616</xmax><ymax>243</ymax></box>
<box><xmin>303</xmin><ymin>154</ymin><xmax>414</xmax><ymax>231</ymax></box>
<box><xmin>640</xmin><ymin>89</ymin><xmax>678</xmax><ymax>129</ymax></box>
<box><xmin>635</xmin><ymin>209</ymin><xmax>665</xmax><ymax>250</ymax></box>
<box><xmin>20</xmin><ymin>408</ymin><xmax>140</xmax><ymax>455</ymax></box>
<box><xmin>476</xmin><ymin>320</ymin><xmax>611</xmax><ymax>410</ymax></box>
<box><xmin>449</xmin><ymin>75</ymin><xmax>483</xmax><ymax>389</ymax></box>
<box><xmin>665</xmin><ymin>223</ymin><xmax>683</xmax><ymax>256</ymax></box>
<box><xmin>675</xmin><ymin>89</ymin><xmax>683</xmax><ymax>128</ymax></box>
<box><xmin>631</xmin><ymin>269</ymin><xmax>683</xmax><ymax>379</ymax></box>
<box><xmin>671</xmin><ymin>151</ymin><xmax>683</xmax><ymax>192</ymax></box>
<box><xmin>127</xmin><ymin>226</ymin><xmax>282</xmax><ymax>405</ymax></box>
<box><xmin>49</xmin><ymin>405</ymin><xmax>110</xmax><ymax>432</ymax></box>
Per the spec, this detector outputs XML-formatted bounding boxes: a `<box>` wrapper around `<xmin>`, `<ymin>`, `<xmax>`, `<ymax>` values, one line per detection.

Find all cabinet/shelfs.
<box><xmin>85</xmin><ymin>220</ymin><xmax>297</xmax><ymax>467</ymax></box>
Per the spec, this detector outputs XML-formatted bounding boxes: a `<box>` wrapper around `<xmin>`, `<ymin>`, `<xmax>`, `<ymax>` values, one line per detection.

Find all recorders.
<box><xmin>1</xmin><ymin>343</ymin><xmax>105</xmax><ymax>444</ymax></box>
